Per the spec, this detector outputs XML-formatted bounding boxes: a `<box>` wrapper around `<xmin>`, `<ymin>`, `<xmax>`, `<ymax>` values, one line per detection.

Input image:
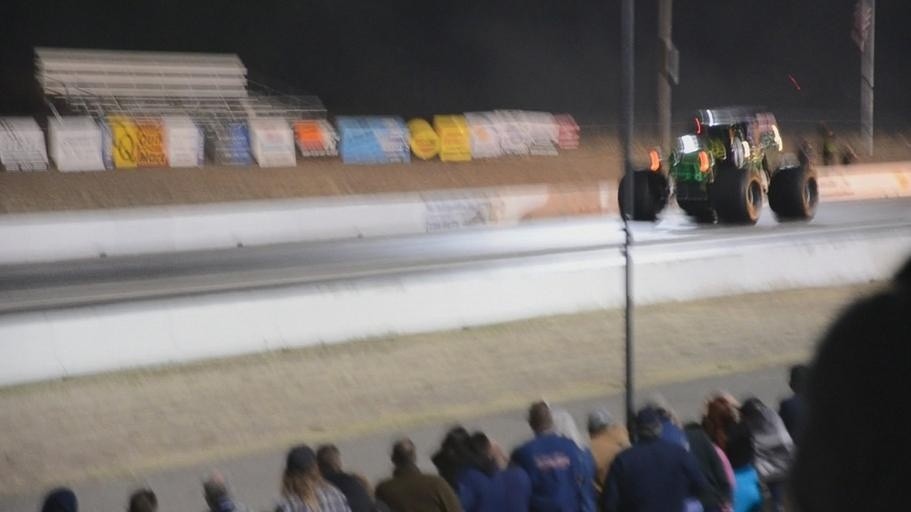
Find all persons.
<box><xmin>320</xmin><ymin>360</ymin><xmax>810</xmax><ymax>512</ymax></box>
<box><xmin>842</xmin><ymin>136</ymin><xmax>857</xmax><ymax>164</ymax></box>
<box><xmin>818</xmin><ymin>119</ymin><xmax>840</xmax><ymax>165</ymax></box>
<box><xmin>127</xmin><ymin>489</ymin><xmax>159</xmax><ymax>512</ymax></box>
<box><xmin>202</xmin><ymin>467</ymin><xmax>238</xmax><ymax>512</ymax></box>
<box><xmin>42</xmin><ymin>486</ymin><xmax>78</xmax><ymax>511</ymax></box>
<box><xmin>798</xmin><ymin>132</ymin><xmax>814</xmax><ymax>167</ymax></box>
<box><xmin>276</xmin><ymin>442</ymin><xmax>356</xmax><ymax>512</ymax></box>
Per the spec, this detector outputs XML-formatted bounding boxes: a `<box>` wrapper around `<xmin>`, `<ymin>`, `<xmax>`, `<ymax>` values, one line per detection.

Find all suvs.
<box><xmin>618</xmin><ymin>104</ymin><xmax>819</xmax><ymax>229</ymax></box>
